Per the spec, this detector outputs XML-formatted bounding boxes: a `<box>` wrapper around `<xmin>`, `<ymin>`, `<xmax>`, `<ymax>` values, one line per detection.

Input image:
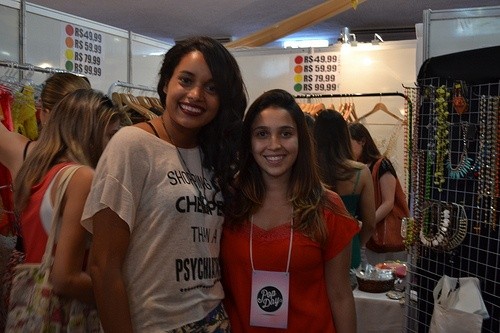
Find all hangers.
<box><xmin>0</xmin><ymin>59</ymin><xmax>35</xmax><ymax>94</ymax></box>
<box><xmin>42</xmin><ymin>67</ymin><xmax>66</xmax><ymax>85</ymax></box>
<box><xmin>111</xmin><ymin>80</ymin><xmax>165</xmax><ymax>126</ymax></box>
<box><xmin>295</xmin><ymin>92</ymin><xmax>408</xmax><ymax>126</ymax></box>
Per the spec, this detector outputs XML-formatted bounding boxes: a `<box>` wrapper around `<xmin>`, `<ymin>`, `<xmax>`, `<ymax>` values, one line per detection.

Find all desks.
<box><xmin>351</xmin><ymin>282</ymin><xmax>405</xmax><ymax>333</ymax></box>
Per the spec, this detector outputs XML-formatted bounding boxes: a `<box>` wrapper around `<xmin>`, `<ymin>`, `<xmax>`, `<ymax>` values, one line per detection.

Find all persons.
<box><xmin>0</xmin><ymin>35</ymin><xmax>410</xmax><ymax>333</ymax></box>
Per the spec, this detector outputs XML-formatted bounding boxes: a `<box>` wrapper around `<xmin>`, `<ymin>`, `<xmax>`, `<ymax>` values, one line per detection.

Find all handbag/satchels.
<box><xmin>6</xmin><ymin>166</ymin><xmax>100</xmax><ymax>333</ymax></box>
<box><xmin>366</xmin><ymin>156</ymin><xmax>409</xmax><ymax>253</ymax></box>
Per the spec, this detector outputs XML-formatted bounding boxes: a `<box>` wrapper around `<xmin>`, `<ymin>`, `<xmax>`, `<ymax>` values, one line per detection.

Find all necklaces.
<box><xmin>161</xmin><ymin>115</ymin><xmax>209</xmax><ymax>213</ymax></box>
<box><xmin>402</xmin><ymin>80</ymin><xmax>500</xmax><ymax>259</ymax></box>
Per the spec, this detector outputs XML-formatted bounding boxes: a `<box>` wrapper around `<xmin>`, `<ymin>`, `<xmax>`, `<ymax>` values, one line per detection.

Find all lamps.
<box><xmin>370</xmin><ymin>33</ymin><xmax>384</xmax><ymax>45</ymax></box>
<box><xmin>340</xmin><ymin>26</ymin><xmax>359</xmax><ymax>48</ymax></box>
